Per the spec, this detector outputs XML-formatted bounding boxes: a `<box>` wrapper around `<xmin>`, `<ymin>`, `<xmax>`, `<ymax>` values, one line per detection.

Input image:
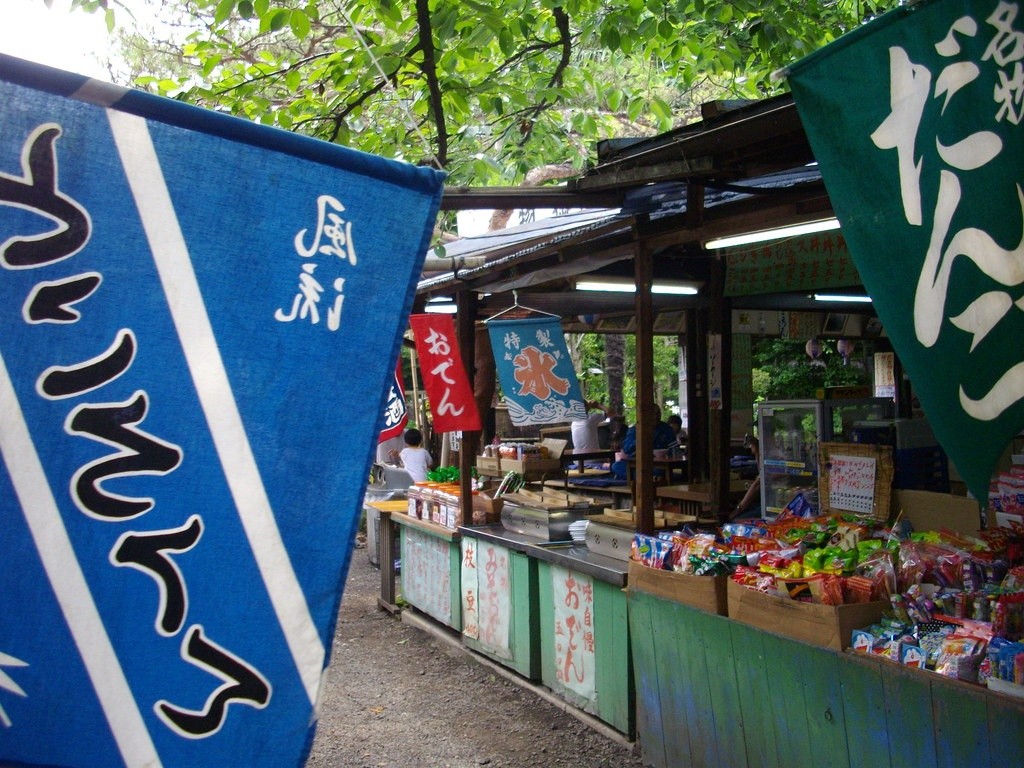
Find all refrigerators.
<box><xmin>758</xmin><ymin>399</ymin><xmax>833</xmax><ymax>521</ymax></box>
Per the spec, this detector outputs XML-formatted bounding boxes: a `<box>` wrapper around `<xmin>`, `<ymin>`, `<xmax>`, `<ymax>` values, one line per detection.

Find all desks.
<box><xmin>623</xmin><ymin>457</ymin><xmax>688</xmax><ymax>485</ymax></box>
<box><xmin>660</xmin><ymin>479</ymin><xmax>754</xmax><ymax>518</ymax></box>
<box><xmin>368</xmin><ymin>500</ymin><xmax>408</xmax><ymax>614</ymax></box>
<box><xmin>565</xmin><ymin>447</ymin><xmax>622</xmax><ymax>472</ymax></box>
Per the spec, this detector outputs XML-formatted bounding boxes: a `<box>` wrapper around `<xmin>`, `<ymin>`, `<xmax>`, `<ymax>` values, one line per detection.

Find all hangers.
<box><xmin>482</xmin><ymin>290</ymin><xmax>563</xmax><ymax>324</ymax></box>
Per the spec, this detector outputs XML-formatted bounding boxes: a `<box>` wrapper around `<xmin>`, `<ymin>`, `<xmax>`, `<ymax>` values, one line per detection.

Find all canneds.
<box><xmin>774</xmin><ymin>486</ymin><xmax>819</xmax><ymax>515</ymax></box>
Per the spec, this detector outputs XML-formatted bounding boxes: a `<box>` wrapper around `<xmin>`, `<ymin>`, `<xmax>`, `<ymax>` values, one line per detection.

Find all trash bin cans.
<box><xmin>362</xmin><ymin>463</ymin><xmax>414</xmax><ymax>565</ymax></box>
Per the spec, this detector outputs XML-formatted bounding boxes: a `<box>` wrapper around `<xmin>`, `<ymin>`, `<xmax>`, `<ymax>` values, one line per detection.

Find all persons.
<box><xmin>374</xmin><ymin>415</ymin><xmax>434</xmax><ymax>488</ymax></box>
<box><xmin>570</xmin><ymin>394</ymin><xmax>686</xmax><ymax>482</ymax></box>
<box><xmin>725</xmin><ymin>414</ymin><xmax>818</xmax><ymax>524</ymax></box>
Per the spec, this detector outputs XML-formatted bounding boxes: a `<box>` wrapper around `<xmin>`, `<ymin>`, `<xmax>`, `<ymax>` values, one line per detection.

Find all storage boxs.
<box><xmin>725</xmin><ymin>574</ymin><xmax>888</xmax><ymax>654</ymax></box>
<box><xmin>472</xmin><ymin>487</ymin><xmax>503</xmax><ymax>524</ymax></box>
<box><xmin>501</xmin><ymin>437</ymin><xmax>569</xmax><ymax>478</ymax></box>
<box><xmin>626</xmin><ymin>555</ymin><xmax>728</xmax><ymax>615</ymax></box>
<box><xmin>476</xmin><ymin>455</ymin><xmax>502</xmax><ymax>477</ymax></box>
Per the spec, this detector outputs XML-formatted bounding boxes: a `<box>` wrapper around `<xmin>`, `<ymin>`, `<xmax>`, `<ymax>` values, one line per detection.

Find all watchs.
<box><xmin>737</xmin><ymin>504</ymin><xmax>742</xmax><ymax>512</ymax></box>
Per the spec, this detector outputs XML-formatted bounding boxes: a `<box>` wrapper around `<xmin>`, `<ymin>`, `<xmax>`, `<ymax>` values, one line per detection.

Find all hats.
<box><xmin>747</xmin><ymin>414</ymin><xmax>783</xmax><ymax>430</ymax></box>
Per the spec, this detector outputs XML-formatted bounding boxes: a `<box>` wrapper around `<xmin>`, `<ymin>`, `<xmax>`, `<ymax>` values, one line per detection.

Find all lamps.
<box><xmin>573</xmin><ymin>275</ymin><xmax>701</xmax><ymax>294</ymax></box>
<box><xmin>806</xmin><ymin>293</ymin><xmax>872</xmax><ymax>302</ymax></box>
<box><xmin>700</xmin><ymin>208</ymin><xmax>840</xmax><ymax>249</ymax></box>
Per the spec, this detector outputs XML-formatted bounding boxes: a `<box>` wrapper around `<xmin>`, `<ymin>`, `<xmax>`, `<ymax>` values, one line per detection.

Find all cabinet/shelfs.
<box><xmin>757</xmin><ymin>396</ymin><xmax>894</xmax><ymax>522</ymax></box>
